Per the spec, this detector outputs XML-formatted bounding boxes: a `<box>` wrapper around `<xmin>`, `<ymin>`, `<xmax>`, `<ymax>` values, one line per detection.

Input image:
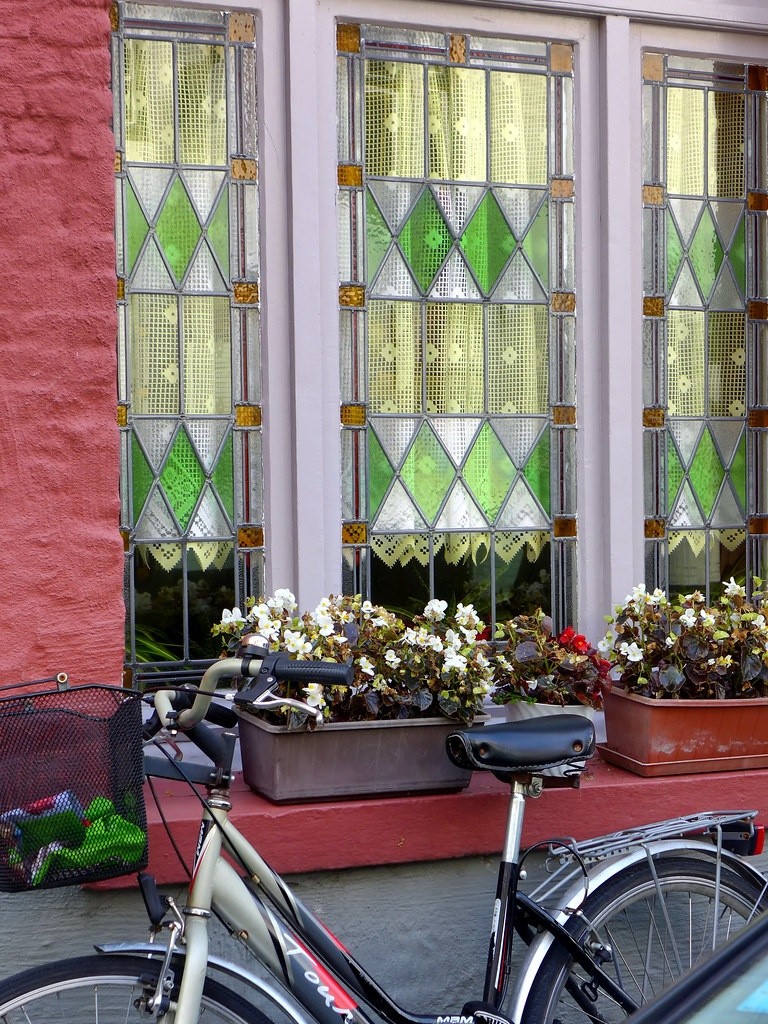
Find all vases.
<box><xmin>224</xmin><ymin>707</ymin><xmax>469</xmax><ymax>805</ymax></box>
<box><xmin>505</xmin><ymin>699</ymin><xmax>593</xmax><ymax>778</ymax></box>
<box><xmin>596</xmin><ymin>682</ymin><xmax>767</xmax><ymax>774</ymax></box>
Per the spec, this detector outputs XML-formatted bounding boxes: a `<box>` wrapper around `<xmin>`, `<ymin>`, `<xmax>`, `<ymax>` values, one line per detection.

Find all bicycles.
<box><xmin>0</xmin><ymin>649</ymin><xmax>768</xmax><ymax>1024</ymax></box>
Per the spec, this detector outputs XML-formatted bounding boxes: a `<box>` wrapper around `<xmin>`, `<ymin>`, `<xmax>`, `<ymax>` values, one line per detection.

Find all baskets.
<box><xmin>0</xmin><ymin>674</ymin><xmax>149</xmax><ymax>893</ymax></box>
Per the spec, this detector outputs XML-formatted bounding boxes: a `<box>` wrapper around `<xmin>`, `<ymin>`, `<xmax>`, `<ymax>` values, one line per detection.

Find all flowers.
<box><xmin>498</xmin><ymin>611</ymin><xmax>607</xmax><ymax>707</ymax></box>
<box><xmin>610</xmin><ymin>580</ymin><xmax>767</xmax><ymax>697</ymax></box>
<box><xmin>217</xmin><ymin>590</ymin><xmax>494</xmax><ymax>721</ymax></box>
